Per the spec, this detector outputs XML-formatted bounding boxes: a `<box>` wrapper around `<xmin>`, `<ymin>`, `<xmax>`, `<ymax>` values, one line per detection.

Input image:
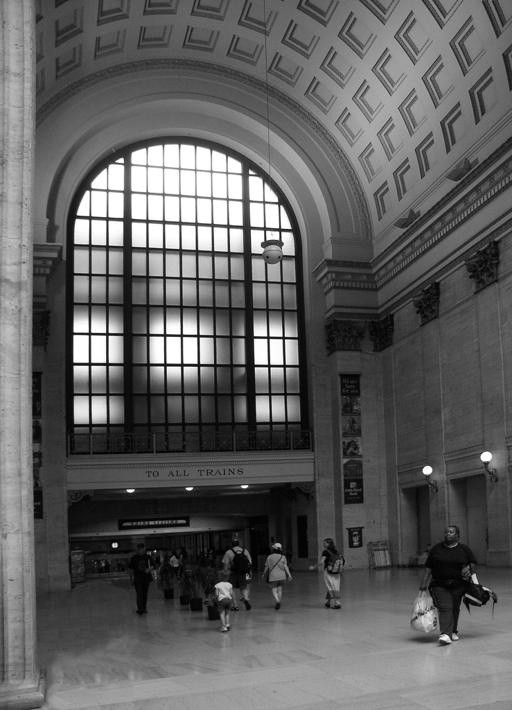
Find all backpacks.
<box><xmin>325</xmin><ymin>550</ymin><xmax>344</xmax><ymax>574</ymax></box>
<box><xmin>464</xmin><ymin>563</ymin><xmax>497</xmax><ymax>607</ymax></box>
<box><xmin>231</xmin><ymin>548</ymin><xmax>250</xmax><ymax>572</ymax></box>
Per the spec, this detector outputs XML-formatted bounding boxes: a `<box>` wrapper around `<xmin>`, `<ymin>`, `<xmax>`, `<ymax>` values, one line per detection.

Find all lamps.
<box><xmin>421</xmin><ymin>465</ymin><xmax>438</xmax><ymax>494</ymax></box>
<box><xmin>260</xmin><ymin>0</ymin><xmax>285</xmax><ymax>264</ymax></box>
<box><xmin>480</xmin><ymin>450</ymin><xmax>498</xmax><ymax>484</ymax></box>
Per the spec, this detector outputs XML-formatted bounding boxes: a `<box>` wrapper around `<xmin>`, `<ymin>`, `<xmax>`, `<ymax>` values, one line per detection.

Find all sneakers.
<box><xmin>135</xmin><ymin>610</ymin><xmax>148</xmax><ymax>615</ymax></box>
<box><xmin>275</xmin><ymin>601</ymin><xmax>281</xmax><ymax>610</ymax></box>
<box><xmin>438</xmin><ymin>633</ymin><xmax>460</xmax><ymax>643</ymax></box>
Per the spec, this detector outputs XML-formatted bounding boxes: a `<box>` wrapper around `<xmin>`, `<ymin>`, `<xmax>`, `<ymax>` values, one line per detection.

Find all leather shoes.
<box><xmin>325</xmin><ymin>602</ymin><xmax>342</xmax><ymax>610</ymax></box>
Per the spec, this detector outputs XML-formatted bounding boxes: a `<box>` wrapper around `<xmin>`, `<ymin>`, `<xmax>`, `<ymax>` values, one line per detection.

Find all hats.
<box><xmin>272</xmin><ymin>543</ymin><xmax>283</xmax><ymax>551</ymax></box>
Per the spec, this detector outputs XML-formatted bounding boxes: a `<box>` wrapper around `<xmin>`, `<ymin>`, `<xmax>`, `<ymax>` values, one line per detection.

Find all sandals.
<box><xmin>231</xmin><ymin>606</ymin><xmax>240</xmax><ymax>611</ymax></box>
<box><xmin>242</xmin><ymin>600</ymin><xmax>252</xmax><ymax>610</ymax></box>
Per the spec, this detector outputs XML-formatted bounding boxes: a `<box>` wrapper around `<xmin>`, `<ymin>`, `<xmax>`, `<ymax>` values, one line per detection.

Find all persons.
<box><xmin>307</xmin><ymin>537</ymin><xmax>345</xmax><ymax>610</ymax></box>
<box><xmin>128</xmin><ymin>537</ymin><xmax>218</xmax><ymax>615</ymax></box>
<box><xmin>221</xmin><ymin>537</ymin><xmax>254</xmax><ymax>612</ymax></box>
<box><xmin>262</xmin><ymin>542</ymin><xmax>293</xmax><ymax>610</ymax></box>
<box><xmin>419</xmin><ymin>525</ymin><xmax>479</xmax><ymax>645</ymax></box>
<box><xmin>215</xmin><ymin>574</ymin><xmax>234</xmax><ymax>633</ymax></box>
<box><xmin>345</xmin><ymin>441</ymin><xmax>360</xmax><ymax>456</ymax></box>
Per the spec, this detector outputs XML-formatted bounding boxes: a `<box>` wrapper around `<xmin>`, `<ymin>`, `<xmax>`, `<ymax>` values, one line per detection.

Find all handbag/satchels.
<box><xmin>266</xmin><ymin>555</ymin><xmax>282</xmax><ymax>584</ymax></box>
<box><xmin>145</xmin><ymin>558</ymin><xmax>159</xmax><ymax>582</ymax></box>
<box><xmin>410</xmin><ymin>588</ymin><xmax>441</xmax><ymax>633</ymax></box>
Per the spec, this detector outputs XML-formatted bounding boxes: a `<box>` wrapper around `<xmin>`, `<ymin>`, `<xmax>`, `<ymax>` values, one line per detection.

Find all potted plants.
<box><xmin>159</xmin><ymin>561</ymin><xmax>220</xmax><ymax>622</ymax></box>
